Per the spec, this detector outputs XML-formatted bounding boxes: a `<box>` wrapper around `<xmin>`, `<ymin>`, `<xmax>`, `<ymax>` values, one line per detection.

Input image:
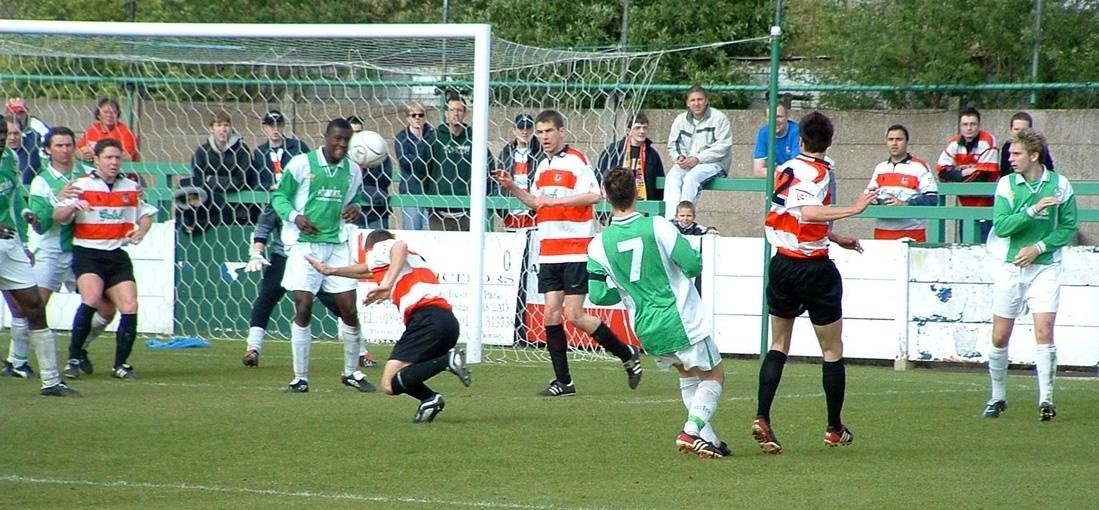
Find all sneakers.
<box><xmin>622</xmin><ymin>344</ymin><xmax>642</xmax><ymax>389</ymax></box>
<box><xmin>241</xmin><ymin>348</ymin><xmax>260</xmax><ymax>367</ymax></box>
<box><xmin>340</xmin><ymin>370</ymin><xmax>376</xmax><ymax>393</ymax></box>
<box><xmin>2</xmin><ymin>356</ymin><xmax>35</xmax><ymax>379</ymax></box>
<box><xmin>447</xmin><ymin>347</ymin><xmax>473</xmax><ymax>388</ymax></box>
<box><xmin>112</xmin><ymin>362</ymin><xmax>140</xmax><ymax>381</ymax></box>
<box><xmin>282</xmin><ymin>373</ymin><xmax>313</xmax><ymax>393</ymax></box>
<box><xmin>674</xmin><ymin>430</ymin><xmax>722</xmax><ymax>465</ymax></box>
<box><xmin>980</xmin><ymin>397</ymin><xmax>1011</xmax><ymax>418</ymax></box>
<box><xmin>823</xmin><ymin>423</ymin><xmax>857</xmax><ymax>449</ymax></box>
<box><xmin>1036</xmin><ymin>399</ymin><xmax>1058</xmax><ymax>421</ymax></box>
<box><xmin>749</xmin><ymin>416</ymin><xmax>785</xmax><ymax>455</ymax></box>
<box><xmin>413</xmin><ymin>392</ymin><xmax>446</xmax><ymax>423</ymax></box>
<box><xmin>703</xmin><ymin>440</ymin><xmax>734</xmax><ymax>455</ymax></box>
<box><xmin>356</xmin><ymin>348</ymin><xmax>378</xmax><ymax>368</ymax></box>
<box><xmin>63</xmin><ymin>359</ymin><xmax>78</xmax><ymax>377</ymax></box>
<box><xmin>536</xmin><ymin>379</ymin><xmax>577</xmax><ymax>396</ymax></box>
<box><xmin>72</xmin><ymin>348</ymin><xmax>96</xmax><ymax>375</ymax></box>
<box><xmin>38</xmin><ymin>380</ymin><xmax>80</xmax><ymax>398</ymax></box>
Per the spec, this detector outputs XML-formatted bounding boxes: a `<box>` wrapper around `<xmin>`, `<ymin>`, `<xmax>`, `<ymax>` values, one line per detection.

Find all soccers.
<box><xmin>347</xmin><ymin>129</ymin><xmax>390</xmax><ymax>169</ymax></box>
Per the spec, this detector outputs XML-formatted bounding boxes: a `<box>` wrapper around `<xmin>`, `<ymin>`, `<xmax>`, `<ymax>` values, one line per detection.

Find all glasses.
<box><xmin>410</xmin><ymin>111</ymin><xmax>428</xmax><ymax>120</ymax></box>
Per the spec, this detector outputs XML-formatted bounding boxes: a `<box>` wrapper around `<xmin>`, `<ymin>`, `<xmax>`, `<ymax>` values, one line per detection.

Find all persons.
<box><xmin>394</xmin><ymin>102</ymin><xmax>435</xmax><ymax>228</ymax></box>
<box><xmin>586</xmin><ymin>169</ymin><xmax>732</xmax><ymax>461</ymax></box>
<box><xmin>242</xmin><ymin>188</ymin><xmax>377</xmax><ymax>370</ymax></box>
<box><xmin>0</xmin><ymin>187</ymin><xmax>38</xmax><ymax>380</ymax></box>
<box><xmin>54</xmin><ymin>139</ymin><xmax>157</xmax><ymax>382</ymax></box>
<box><xmin>74</xmin><ymin>98</ymin><xmax>142</xmax><ymax>187</ymax></box>
<box><xmin>754</xmin><ymin>99</ymin><xmax>802</xmax><ymax>183</ymax></box>
<box><xmin>665</xmin><ymin>201</ymin><xmax>720</xmax><ymax>237</ymax></box>
<box><xmin>272</xmin><ymin>116</ymin><xmax>375</xmax><ymax>392</ymax></box>
<box><xmin>303</xmin><ymin>230</ymin><xmax>472</xmax><ymax>424</ymax></box>
<box><xmin>490</xmin><ymin>111</ymin><xmax>641</xmax><ymax>398</ymax></box>
<box><xmin>0</xmin><ymin>95</ymin><xmax>52</xmax><ymax>155</ymax></box>
<box><xmin>663</xmin><ymin>85</ymin><xmax>731</xmax><ymax>223</ymax></box>
<box><xmin>982</xmin><ymin>129</ymin><xmax>1079</xmax><ymax>421</ymax></box>
<box><xmin>494</xmin><ymin>115</ymin><xmax>549</xmax><ymax>232</ymax></box>
<box><xmin>3</xmin><ymin>120</ymin><xmax>46</xmax><ymax>193</ymax></box>
<box><xmin>191</xmin><ymin>111</ymin><xmax>250</xmax><ymax>223</ymax></box>
<box><xmin>750</xmin><ymin>114</ymin><xmax>881</xmax><ymax>453</ymax></box>
<box><xmin>595</xmin><ymin>114</ymin><xmax>663</xmax><ymax>200</ymax></box>
<box><xmin>249</xmin><ymin>111</ymin><xmax>311</xmax><ymax>190</ymax></box>
<box><xmin>863</xmin><ymin>124</ymin><xmax>938</xmax><ymax>241</ymax></box>
<box><xmin>936</xmin><ymin>107</ymin><xmax>999</xmax><ymax>245</ymax></box>
<box><xmin>0</xmin><ymin>113</ymin><xmax>84</xmax><ymax>400</ymax></box>
<box><xmin>1000</xmin><ymin>112</ymin><xmax>1055</xmax><ymax>182</ymax></box>
<box><xmin>175</xmin><ymin>178</ymin><xmax>233</xmax><ymax>234</ymax></box>
<box><xmin>427</xmin><ymin>97</ymin><xmax>495</xmax><ymax>231</ymax></box>
<box><xmin>0</xmin><ymin>126</ymin><xmax>142</xmax><ymax>378</ymax></box>
<box><xmin>345</xmin><ymin>114</ymin><xmax>391</xmax><ymax>230</ymax></box>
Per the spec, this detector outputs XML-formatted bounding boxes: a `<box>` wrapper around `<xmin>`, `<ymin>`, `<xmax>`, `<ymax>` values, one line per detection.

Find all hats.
<box><xmin>515</xmin><ymin>113</ymin><xmax>534</xmax><ymax>127</ymax></box>
<box><xmin>2</xmin><ymin>97</ymin><xmax>28</xmax><ymax>114</ymax></box>
<box><xmin>261</xmin><ymin>109</ymin><xmax>287</xmax><ymax>124</ymax></box>
